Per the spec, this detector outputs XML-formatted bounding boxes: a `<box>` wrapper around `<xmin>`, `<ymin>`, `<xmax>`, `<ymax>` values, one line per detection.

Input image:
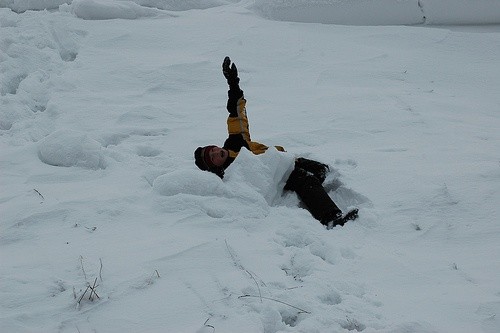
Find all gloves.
<box><xmin>221</xmin><ymin>56</ymin><xmax>241</xmax><ymax>91</ymax></box>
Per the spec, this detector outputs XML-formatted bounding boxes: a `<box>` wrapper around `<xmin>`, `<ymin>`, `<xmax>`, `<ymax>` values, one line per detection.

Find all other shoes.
<box><xmin>326</xmin><ymin>206</ymin><xmax>359</xmax><ymax>230</ymax></box>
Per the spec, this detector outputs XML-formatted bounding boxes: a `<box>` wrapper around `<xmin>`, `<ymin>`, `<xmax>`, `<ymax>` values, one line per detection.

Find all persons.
<box><xmin>194</xmin><ymin>56</ymin><xmax>359</xmax><ymax>230</ymax></box>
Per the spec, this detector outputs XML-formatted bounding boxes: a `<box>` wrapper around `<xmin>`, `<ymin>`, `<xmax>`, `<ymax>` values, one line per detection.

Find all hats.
<box><xmin>193</xmin><ymin>145</ymin><xmax>218</xmax><ymax>176</ymax></box>
